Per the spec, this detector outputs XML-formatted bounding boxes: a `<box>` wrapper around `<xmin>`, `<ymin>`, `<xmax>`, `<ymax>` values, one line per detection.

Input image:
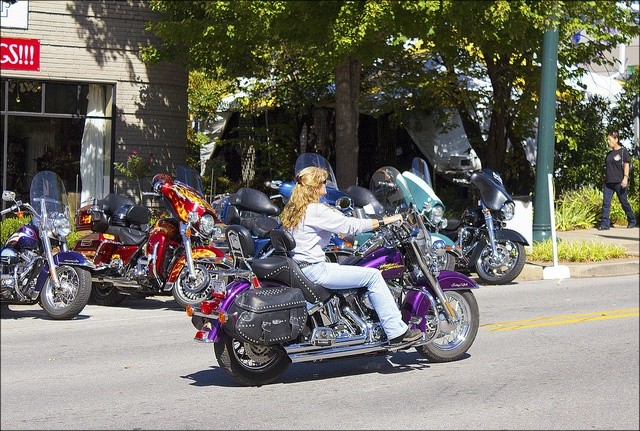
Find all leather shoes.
<box><xmin>390</xmin><ymin>329</ymin><xmax>423</xmax><ymax>345</ymax></box>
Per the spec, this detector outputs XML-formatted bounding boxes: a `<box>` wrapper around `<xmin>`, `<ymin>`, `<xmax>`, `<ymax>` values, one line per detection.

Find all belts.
<box><xmin>298</xmin><ymin>261</ymin><xmax>309</xmax><ymax>269</ymax></box>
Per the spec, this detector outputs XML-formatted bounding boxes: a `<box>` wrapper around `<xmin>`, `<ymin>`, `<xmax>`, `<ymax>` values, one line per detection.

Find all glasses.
<box><xmin>319</xmin><ymin>180</ymin><xmax>326</xmax><ymax>184</ymax></box>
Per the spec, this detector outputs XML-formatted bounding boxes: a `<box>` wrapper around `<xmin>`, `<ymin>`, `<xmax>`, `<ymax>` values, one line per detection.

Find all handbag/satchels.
<box><xmin>223</xmin><ymin>287</ymin><xmax>308</xmax><ymax>346</ymax></box>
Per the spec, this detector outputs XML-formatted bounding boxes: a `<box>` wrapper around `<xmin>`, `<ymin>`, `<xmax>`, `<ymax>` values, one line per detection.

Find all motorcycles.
<box><xmin>411</xmin><ymin>157</ymin><xmax>530</xmax><ymax>286</ymax></box>
<box><xmin>0</xmin><ymin>170</ymin><xmax>96</xmax><ymax>320</ymax></box>
<box><xmin>73</xmin><ymin>180</ymin><xmax>233</xmax><ymax>307</ymax></box>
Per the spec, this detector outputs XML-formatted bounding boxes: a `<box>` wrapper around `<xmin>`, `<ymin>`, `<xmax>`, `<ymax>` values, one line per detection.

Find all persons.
<box><xmin>598</xmin><ymin>131</ymin><xmax>638</xmax><ymax>230</ymax></box>
<box><xmin>280</xmin><ymin>166</ymin><xmax>423</xmax><ymax>347</ymax></box>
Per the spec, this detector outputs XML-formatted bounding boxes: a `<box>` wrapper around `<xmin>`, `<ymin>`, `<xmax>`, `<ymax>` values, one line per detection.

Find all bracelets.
<box><xmin>624</xmin><ymin>175</ymin><xmax>629</xmax><ymax>179</ymax></box>
<box><xmin>376</xmin><ymin>218</ymin><xmax>384</xmax><ymax>229</ymax></box>
